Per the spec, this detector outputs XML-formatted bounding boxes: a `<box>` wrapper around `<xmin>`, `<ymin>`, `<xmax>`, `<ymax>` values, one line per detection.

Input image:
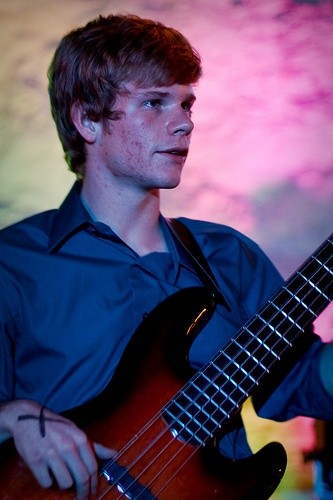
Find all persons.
<box><xmin>1</xmin><ymin>14</ymin><xmax>333</xmax><ymax>500</ymax></box>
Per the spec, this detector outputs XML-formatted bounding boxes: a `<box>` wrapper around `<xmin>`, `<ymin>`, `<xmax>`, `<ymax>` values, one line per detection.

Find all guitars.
<box><xmin>0</xmin><ymin>231</ymin><xmax>333</xmax><ymax>499</ymax></box>
